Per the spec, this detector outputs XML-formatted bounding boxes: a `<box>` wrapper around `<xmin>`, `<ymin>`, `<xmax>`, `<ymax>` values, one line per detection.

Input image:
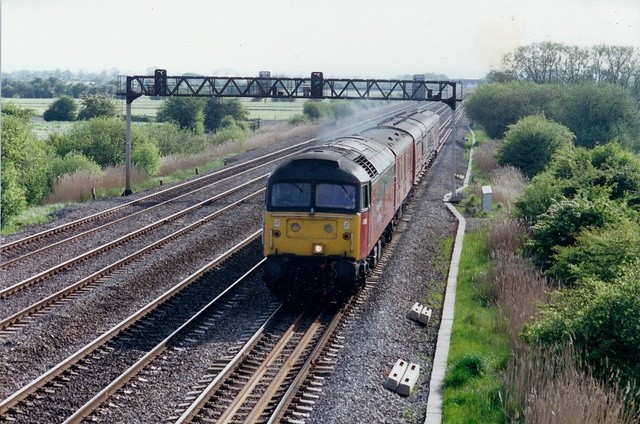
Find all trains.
<box><xmin>262</xmin><ymin>108</ymin><xmax>440</xmax><ymax>288</ymax></box>
